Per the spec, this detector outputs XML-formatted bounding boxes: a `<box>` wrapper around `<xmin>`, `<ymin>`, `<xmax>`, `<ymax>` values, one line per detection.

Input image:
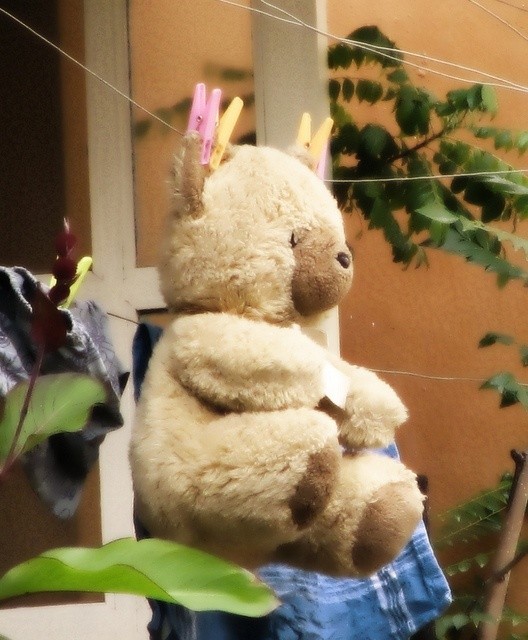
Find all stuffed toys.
<box><xmin>127</xmin><ymin>130</ymin><xmax>430</xmax><ymax>582</ymax></box>
